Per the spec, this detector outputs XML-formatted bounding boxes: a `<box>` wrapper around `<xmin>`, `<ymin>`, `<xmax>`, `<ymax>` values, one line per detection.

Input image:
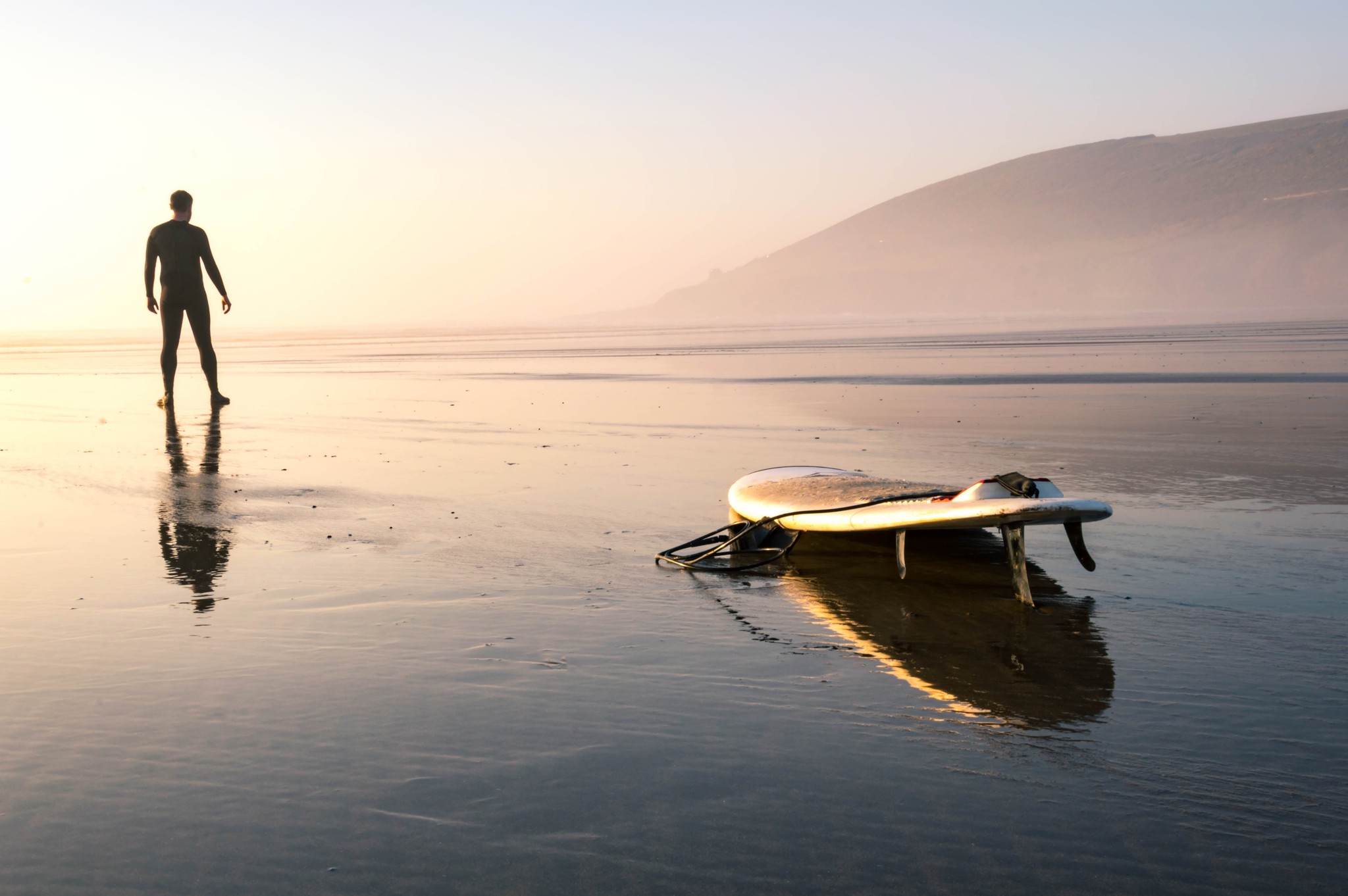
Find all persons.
<box><xmin>144</xmin><ymin>190</ymin><xmax>232</xmax><ymax>405</ymax></box>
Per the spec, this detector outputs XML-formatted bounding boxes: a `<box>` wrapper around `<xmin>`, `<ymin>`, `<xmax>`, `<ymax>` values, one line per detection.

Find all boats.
<box><xmin>724</xmin><ymin>465</ymin><xmax>1112</xmax><ymax>608</ymax></box>
